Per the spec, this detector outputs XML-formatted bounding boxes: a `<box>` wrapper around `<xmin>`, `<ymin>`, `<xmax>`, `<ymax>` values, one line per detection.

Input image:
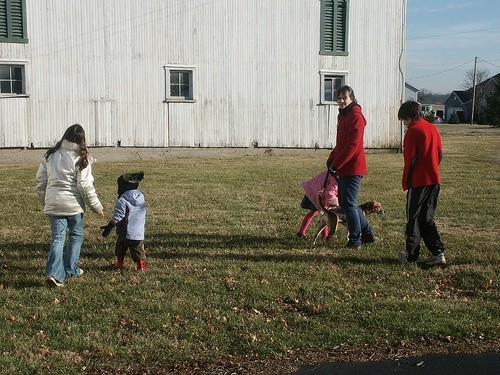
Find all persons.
<box><xmin>398</xmin><ymin>101</ymin><xmax>447</xmax><ymax>266</ymax></box>
<box><xmin>100</xmin><ymin>171</ymin><xmax>147</xmax><ymax>272</ymax></box>
<box><xmin>36</xmin><ymin>124</ymin><xmax>104</xmax><ymax>287</ymax></box>
<box><xmin>296</xmin><ymin>170</ymin><xmax>340</xmax><ymax>240</ymax></box>
<box><xmin>326</xmin><ymin>85</ymin><xmax>377</xmax><ymax>251</ymax></box>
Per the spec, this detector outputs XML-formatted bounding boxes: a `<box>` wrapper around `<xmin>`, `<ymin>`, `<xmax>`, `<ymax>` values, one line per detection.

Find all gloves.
<box><xmin>326</xmin><ymin>160</ymin><xmax>336</xmax><ymax>176</ymax></box>
<box><xmin>100</xmin><ymin>220</ymin><xmax>116</xmax><ymax>237</ymax></box>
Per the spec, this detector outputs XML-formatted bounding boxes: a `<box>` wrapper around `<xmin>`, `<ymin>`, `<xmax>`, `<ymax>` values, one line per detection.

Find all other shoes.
<box><xmin>135</xmin><ymin>259</ymin><xmax>146</xmax><ymax>273</ymax></box>
<box><xmin>344</xmin><ymin>240</ymin><xmax>361</xmax><ymax>249</ymax></box>
<box><xmin>361</xmin><ymin>233</ymin><xmax>375</xmax><ymax>243</ymax></box>
<box><xmin>64</xmin><ymin>267</ymin><xmax>84</xmax><ymax>278</ymax></box>
<box><xmin>111</xmin><ymin>256</ymin><xmax>124</xmax><ymax>270</ymax></box>
<box><xmin>398</xmin><ymin>251</ymin><xmax>417</xmax><ymax>266</ymax></box>
<box><xmin>45</xmin><ymin>272</ymin><xmax>64</xmax><ymax>288</ymax></box>
<box><xmin>425</xmin><ymin>252</ymin><xmax>446</xmax><ymax>265</ymax></box>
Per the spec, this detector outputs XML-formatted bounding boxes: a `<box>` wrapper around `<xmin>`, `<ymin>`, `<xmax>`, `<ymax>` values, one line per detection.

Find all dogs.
<box><xmin>311</xmin><ymin>188</ymin><xmax>386</xmax><ymax>246</ymax></box>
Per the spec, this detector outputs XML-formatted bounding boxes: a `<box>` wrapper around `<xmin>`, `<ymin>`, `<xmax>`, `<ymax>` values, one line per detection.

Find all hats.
<box><xmin>117</xmin><ymin>171</ymin><xmax>144</xmax><ymax>198</ymax></box>
